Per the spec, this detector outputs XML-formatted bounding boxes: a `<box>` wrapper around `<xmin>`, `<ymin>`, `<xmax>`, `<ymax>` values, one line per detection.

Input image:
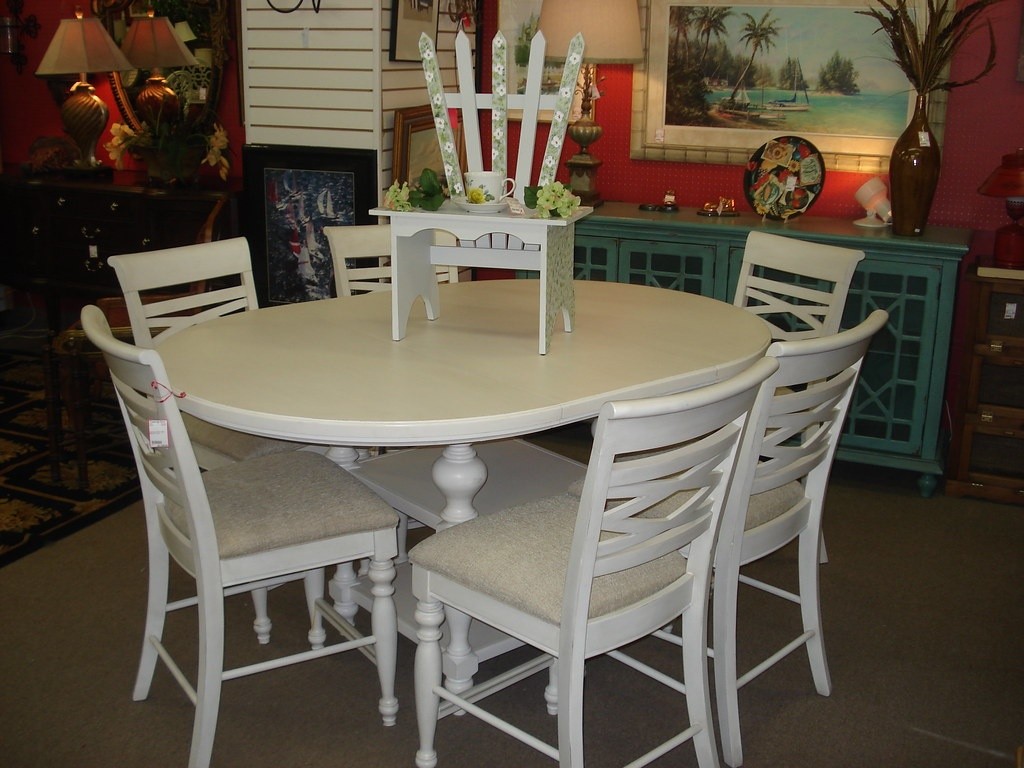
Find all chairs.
<box><xmin>107</xmin><ymin>237</ymin><xmax>363</xmax><ymax>482</ymax></box>
<box><xmin>609</xmin><ymin>231</ymin><xmax>865</xmax><ymax>475</ymax></box>
<box><xmin>79</xmin><ymin>305</ymin><xmax>402</xmax><ymax>768</ymax></box>
<box><xmin>413</xmin><ymin>354</ymin><xmax>780</xmax><ymax>767</ymax></box>
<box><xmin>52</xmin><ymin>197</ymin><xmax>226</xmax><ymax>486</ymax></box>
<box><xmin>569</xmin><ymin>309</ymin><xmax>892</xmax><ymax>762</ymax></box>
<box><xmin>322</xmin><ymin>220</ymin><xmax>459</xmax><ymax>300</ymax></box>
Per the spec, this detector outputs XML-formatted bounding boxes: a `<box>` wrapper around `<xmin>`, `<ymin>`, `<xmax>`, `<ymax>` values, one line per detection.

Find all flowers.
<box><xmin>101</xmin><ymin>90</ymin><xmax>231</xmax><ymax>182</ymax></box>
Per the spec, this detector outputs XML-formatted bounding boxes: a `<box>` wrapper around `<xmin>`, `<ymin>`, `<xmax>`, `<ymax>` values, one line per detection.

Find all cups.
<box><xmin>464</xmin><ymin>171</ymin><xmax>516</xmax><ymax>204</ymax></box>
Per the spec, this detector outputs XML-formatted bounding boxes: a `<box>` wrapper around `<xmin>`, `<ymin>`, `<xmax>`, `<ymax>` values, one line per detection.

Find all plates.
<box><xmin>450</xmin><ymin>196</ymin><xmax>508</xmax><ymax>214</ymax></box>
<box><xmin>743</xmin><ymin>136</ymin><xmax>825</xmax><ymax>220</ymax></box>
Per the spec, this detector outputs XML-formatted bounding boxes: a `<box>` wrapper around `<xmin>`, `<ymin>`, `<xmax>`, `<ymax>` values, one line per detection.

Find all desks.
<box><xmin>152</xmin><ymin>275</ymin><xmax>771</xmax><ymax>719</ymax></box>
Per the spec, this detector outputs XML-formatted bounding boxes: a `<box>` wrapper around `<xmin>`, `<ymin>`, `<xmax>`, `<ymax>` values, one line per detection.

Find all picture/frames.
<box><xmin>389</xmin><ymin>0</ymin><xmax>441</xmax><ymax>63</ymax></box>
<box><xmin>391</xmin><ymin>103</ymin><xmax>458</xmax><ymax>192</ymax></box>
<box><xmin>497</xmin><ymin>0</ymin><xmax>598</xmax><ymax>124</ymax></box>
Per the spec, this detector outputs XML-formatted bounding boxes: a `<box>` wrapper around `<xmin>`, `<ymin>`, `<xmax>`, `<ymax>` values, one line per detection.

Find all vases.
<box><xmin>139</xmin><ymin>144</ymin><xmax>206</xmax><ymax>190</ymax></box>
<box><xmin>889</xmin><ymin>94</ymin><xmax>942</xmax><ymax>237</ymax></box>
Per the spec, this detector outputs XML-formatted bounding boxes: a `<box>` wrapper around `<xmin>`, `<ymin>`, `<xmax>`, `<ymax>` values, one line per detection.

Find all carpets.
<box><xmin>0</xmin><ymin>348</ymin><xmax>142</xmax><ymax>571</ymax></box>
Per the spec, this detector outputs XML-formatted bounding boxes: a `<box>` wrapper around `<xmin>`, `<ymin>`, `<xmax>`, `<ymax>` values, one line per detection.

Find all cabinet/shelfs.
<box><xmin>517</xmin><ymin>224</ymin><xmax>958</xmax><ymax>482</ymax></box>
<box><xmin>0</xmin><ymin>173</ymin><xmax>229</xmax><ymax>301</ymax></box>
<box><xmin>945</xmin><ymin>276</ymin><xmax>1024</xmax><ymax>508</ymax></box>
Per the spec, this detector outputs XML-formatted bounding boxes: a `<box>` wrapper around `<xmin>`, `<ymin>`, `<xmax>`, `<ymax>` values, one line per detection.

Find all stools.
<box><xmin>370</xmin><ymin>198</ymin><xmax>593</xmax><ymax>360</ymax></box>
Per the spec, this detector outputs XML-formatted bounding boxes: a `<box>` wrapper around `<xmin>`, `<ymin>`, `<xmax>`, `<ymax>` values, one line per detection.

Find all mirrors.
<box><xmin>96</xmin><ymin>0</ymin><xmax>228</xmax><ymax>147</ymax></box>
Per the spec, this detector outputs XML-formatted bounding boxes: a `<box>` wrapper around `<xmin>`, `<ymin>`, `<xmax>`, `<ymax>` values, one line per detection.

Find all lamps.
<box><xmin>0</xmin><ymin>0</ymin><xmax>38</xmax><ymax>71</ymax></box>
<box><xmin>34</xmin><ymin>7</ymin><xmax>133</xmax><ymax>171</ymax></box>
<box><xmin>532</xmin><ymin>0</ymin><xmax>645</xmax><ymax>216</ymax></box>
<box><xmin>117</xmin><ymin>4</ymin><xmax>202</xmax><ymax>132</ymax></box>
<box><xmin>977</xmin><ymin>148</ymin><xmax>1024</xmax><ymax>271</ymax></box>
